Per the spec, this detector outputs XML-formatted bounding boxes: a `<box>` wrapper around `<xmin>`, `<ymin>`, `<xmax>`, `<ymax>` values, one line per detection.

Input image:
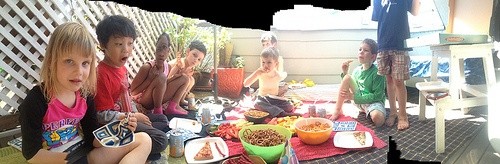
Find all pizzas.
<box><xmin>194</xmin><ymin>142</ymin><xmax>213</xmax><ymax>160</ymax></box>
<box><xmin>353</xmin><ymin>131</ymin><xmax>365</xmax><ymax>145</ymax></box>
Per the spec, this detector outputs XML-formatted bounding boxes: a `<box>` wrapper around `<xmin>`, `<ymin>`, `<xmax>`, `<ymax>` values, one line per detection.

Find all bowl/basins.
<box><xmin>244</xmin><ymin>113</ymin><xmax>269</xmax><ymax>123</ymax></box>
<box><xmin>239</xmin><ymin>124</ymin><xmax>292</xmax><ymax>162</ymax></box>
<box><xmin>294</xmin><ymin>118</ymin><xmax>333</xmax><ymax>145</ymax></box>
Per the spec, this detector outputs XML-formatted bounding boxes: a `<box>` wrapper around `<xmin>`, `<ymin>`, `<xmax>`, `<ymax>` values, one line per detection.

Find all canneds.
<box><xmin>169</xmin><ymin>131</ymin><xmax>184</xmax><ymax>157</ymax></box>
<box><xmin>202</xmin><ymin>108</ymin><xmax>211</xmax><ymax>125</ymax></box>
<box><xmin>317</xmin><ymin>108</ymin><xmax>326</xmax><ymax>118</ymax></box>
<box><xmin>188</xmin><ymin>97</ymin><xmax>195</xmax><ymax>110</ymax></box>
<box><xmin>308</xmin><ymin>105</ymin><xmax>316</xmax><ymax>118</ymax></box>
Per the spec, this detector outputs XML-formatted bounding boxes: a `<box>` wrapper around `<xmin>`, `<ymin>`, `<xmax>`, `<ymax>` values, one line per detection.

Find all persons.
<box><xmin>327</xmin><ymin>38</ymin><xmax>386</xmax><ymax>127</ymax></box>
<box><xmin>19</xmin><ymin>14</ymin><xmax>206</xmax><ymax>164</ymax></box>
<box><xmin>260</xmin><ymin>34</ymin><xmax>288</xmax><ymax>96</ymax></box>
<box><xmin>372</xmin><ymin>0</ymin><xmax>420</xmax><ymax>130</ymax></box>
<box><xmin>243</xmin><ymin>47</ymin><xmax>292</xmax><ymax>116</ymax></box>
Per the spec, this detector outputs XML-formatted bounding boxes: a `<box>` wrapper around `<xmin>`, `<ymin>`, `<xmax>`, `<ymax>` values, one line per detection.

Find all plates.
<box><xmin>169</xmin><ymin>118</ymin><xmax>202</xmax><ymax>133</ymax></box>
<box><xmin>185</xmin><ymin>137</ymin><xmax>228</xmax><ymax>164</ymax></box>
<box><xmin>416</xmin><ymin>81</ymin><xmax>448</xmax><ymax>91</ymax></box>
<box><xmin>334</xmin><ymin>131</ymin><xmax>374</xmax><ymax>148</ymax></box>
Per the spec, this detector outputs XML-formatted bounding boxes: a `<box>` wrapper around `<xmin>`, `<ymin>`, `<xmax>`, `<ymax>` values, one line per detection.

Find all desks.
<box><xmin>416</xmin><ymin>43</ymin><xmax>497</xmax><ymax>154</ymax></box>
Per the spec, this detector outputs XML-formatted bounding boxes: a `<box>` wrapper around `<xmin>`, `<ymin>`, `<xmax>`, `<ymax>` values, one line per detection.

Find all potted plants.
<box><xmin>161</xmin><ymin>13</ymin><xmax>231</xmax><ymax>86</ymax></box>
<box><xmin>217</xmin><ymin>54</ymin><xmax>246</xmax><ymax>98</ymax></box>
